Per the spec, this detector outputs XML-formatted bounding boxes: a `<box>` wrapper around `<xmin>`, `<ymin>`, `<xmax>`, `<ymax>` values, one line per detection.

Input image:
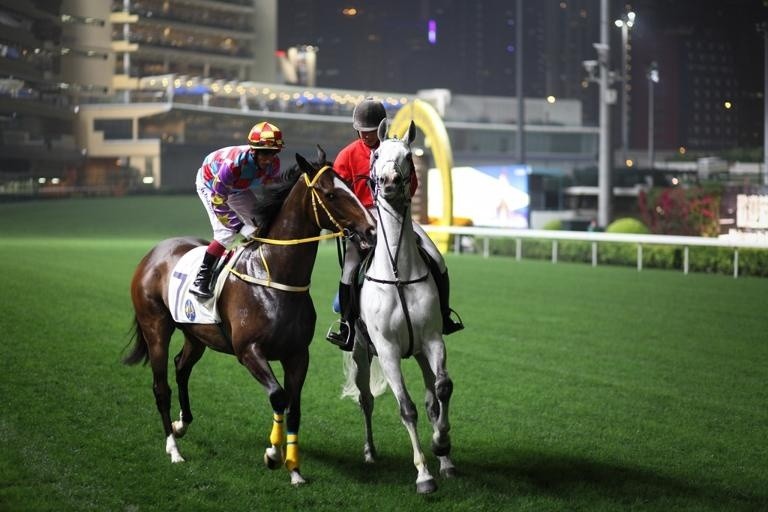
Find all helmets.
<box><xmin>247</xmin><ymin>120</ymin><xmax>284</xmax><ymax>152</ymax></box>
<box><xmin>350</xmin><ymin>96</ymin><xmax>387</xmax><ymax>134</ymax></box>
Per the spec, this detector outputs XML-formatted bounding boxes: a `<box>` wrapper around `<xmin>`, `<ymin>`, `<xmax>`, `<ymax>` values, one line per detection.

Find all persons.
<box><xmin>327</xmin><ymin>97</ymin><xmax>462</xmax><ymax>342</ymax></box>
<box><xmin>188</xmin><ymin>122</ymin><xmax>285</xmax><ymax>299</ymax></box>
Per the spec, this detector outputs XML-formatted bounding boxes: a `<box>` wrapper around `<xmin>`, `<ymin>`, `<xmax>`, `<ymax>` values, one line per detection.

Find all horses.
<box><xmin>338</xmin><ymin>117</ymin><xmax>457</xmax><ymax>495</ymax></box>
<box><xmin>119</xmin><ymin>143</ymin><xmax>377</xmax><ymax>488</ymax></box>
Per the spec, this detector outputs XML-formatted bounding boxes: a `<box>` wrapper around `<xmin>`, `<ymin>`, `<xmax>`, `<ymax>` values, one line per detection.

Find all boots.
<box><xmin>327</xmin><ymin>280</ymin><xmax>359</xmax><ymax>352</ymax></box>
<box><xmin>434</xmin><ymin>266</ymin><xmax>466</xmax><ymax>336</ymax></box>
<box><xmin>188</xmin><ymin>251</ymin><xmax>221</xmax><ymax>299</ymax></box>
